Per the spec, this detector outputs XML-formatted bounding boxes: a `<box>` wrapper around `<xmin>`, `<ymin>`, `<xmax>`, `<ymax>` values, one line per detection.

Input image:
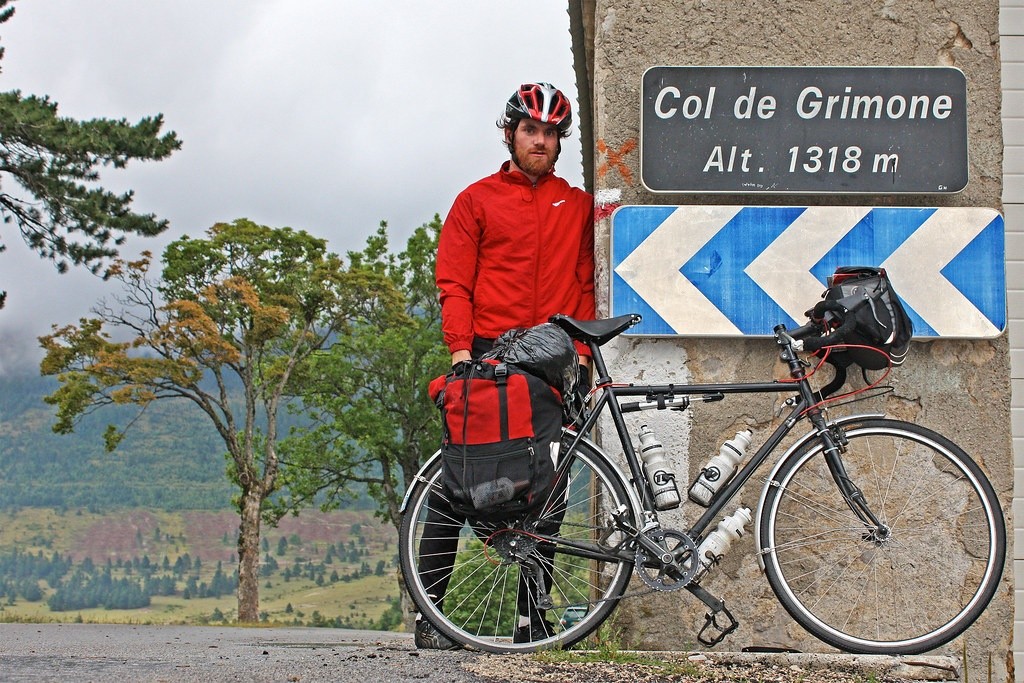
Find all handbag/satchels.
<box><xmin>479</xmin><ymin>322</ymin><xmax>579</xmax><ymax>399</ymax></box>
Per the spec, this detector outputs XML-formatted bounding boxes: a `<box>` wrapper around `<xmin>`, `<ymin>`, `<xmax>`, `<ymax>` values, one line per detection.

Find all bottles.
<box><xmin>683</xmin><ymin>507</ymin><xmax>752</xmax><ymax>582</ymax></box>
<box><xmin>638</xmin><ymin>424</ymin><xmax>682</xmax><ymax>508</ymax></box>
<box><xmin>689</xmin><ymin>429</ymin><xmax>753</xmax><ymax>508</ymax></box>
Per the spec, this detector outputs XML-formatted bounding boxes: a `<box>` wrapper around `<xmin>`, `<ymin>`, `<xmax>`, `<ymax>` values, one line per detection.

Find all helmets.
<box><xmin>506</xmin><ymin>82</ymin><xmax>573</xmax><ymax>130</ymax></box>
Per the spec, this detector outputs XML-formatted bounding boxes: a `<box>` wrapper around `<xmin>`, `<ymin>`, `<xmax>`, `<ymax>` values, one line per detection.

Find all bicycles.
<box><xmin>393</xmin><ymin>282</ymin><xmax>1008</xmax><ymax>658</ymax></box>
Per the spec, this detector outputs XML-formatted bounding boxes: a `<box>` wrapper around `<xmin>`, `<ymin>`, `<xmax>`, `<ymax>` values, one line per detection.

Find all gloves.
<box><xmin>578</xmin><ymin>363</ymin><xmax>591</xmax><ymax>398</ymax></box>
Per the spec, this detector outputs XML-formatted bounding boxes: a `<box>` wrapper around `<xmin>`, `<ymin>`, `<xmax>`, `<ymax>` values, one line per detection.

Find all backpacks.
<box><xmin>429</xmin><ymin>360</ymin><xmax>563</xmax><ymax>517</ymax></box>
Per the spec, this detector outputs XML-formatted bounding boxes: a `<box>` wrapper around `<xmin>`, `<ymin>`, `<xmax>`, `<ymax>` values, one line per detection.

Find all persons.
<box><xmin>414</xmin><ymin>83</ymin><xmax>594</xmax><ymax>649</ymax></box>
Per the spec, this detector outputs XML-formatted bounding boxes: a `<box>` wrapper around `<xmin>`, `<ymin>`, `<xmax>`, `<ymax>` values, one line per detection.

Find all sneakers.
<box><xmin>513</xmin><ymin>617</ymin><xmax>557</xmax><ymax>644</ymax></box>
<box><xmin>414</xmin><ymin>608</ymin><xmax>466</xmax><ymax>651</ymax></box>
